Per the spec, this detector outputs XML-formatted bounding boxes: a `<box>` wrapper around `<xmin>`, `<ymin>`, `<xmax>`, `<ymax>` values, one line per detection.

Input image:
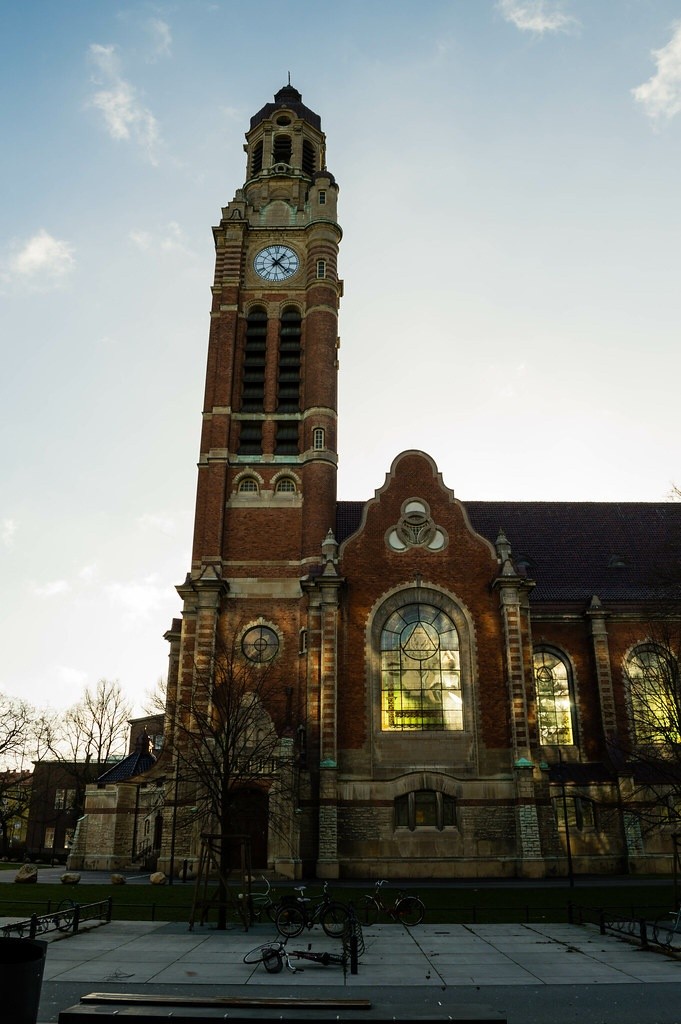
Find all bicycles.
<box><xmin>230</xmin><ymin>875</ymin><xmax>352</xmax><ymax>938</ymax></box>
<box><xmin>355</xmin><ymin>879</ymin><xmax>426</xmax><ymax>926</ymax></box>
<box><xmin>243</xmin><ymin>931</ymin><xmax>345</xmax><ymax>974</ymax></box>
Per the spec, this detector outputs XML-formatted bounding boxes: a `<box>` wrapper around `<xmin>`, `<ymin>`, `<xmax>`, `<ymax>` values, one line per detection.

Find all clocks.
<box><xmin>254</xmin><ymin>245</ymin><xmax>299</xmax><ymax>281</ymax></box>
